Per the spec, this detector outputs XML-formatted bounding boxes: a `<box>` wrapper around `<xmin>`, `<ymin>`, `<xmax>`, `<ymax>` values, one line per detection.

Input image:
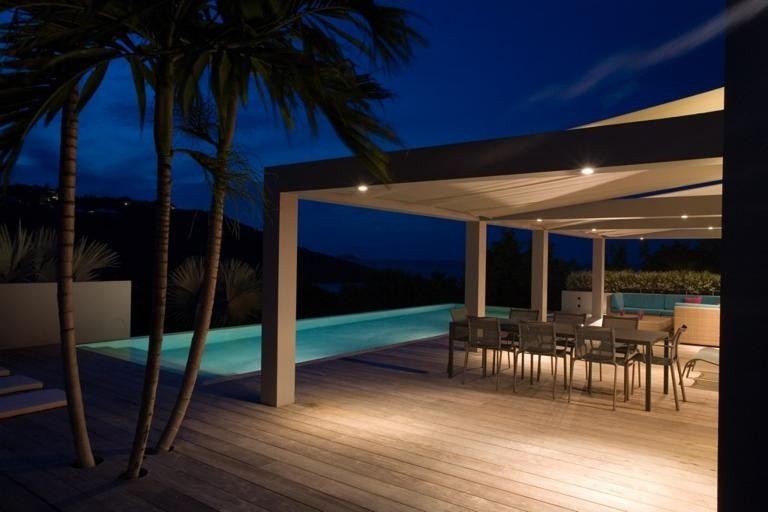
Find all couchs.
<box><xmin>610</xmin><ymin>292</ymin><xmax>720</xmax><ymax>316</ymax></box>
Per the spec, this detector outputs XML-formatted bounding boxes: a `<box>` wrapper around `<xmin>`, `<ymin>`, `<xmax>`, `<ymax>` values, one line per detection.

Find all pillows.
<box><xmin>683</xmin><ymin>297</ymin><xmax>703</xmax><ymax>304</ymax></box>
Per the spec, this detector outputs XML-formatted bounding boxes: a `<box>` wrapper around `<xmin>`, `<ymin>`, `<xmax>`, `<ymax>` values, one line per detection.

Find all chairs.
<box><xmin>445</xmin><ymin>309</ymin><xmax>688</xmax><ymax>413</ymax></box>
